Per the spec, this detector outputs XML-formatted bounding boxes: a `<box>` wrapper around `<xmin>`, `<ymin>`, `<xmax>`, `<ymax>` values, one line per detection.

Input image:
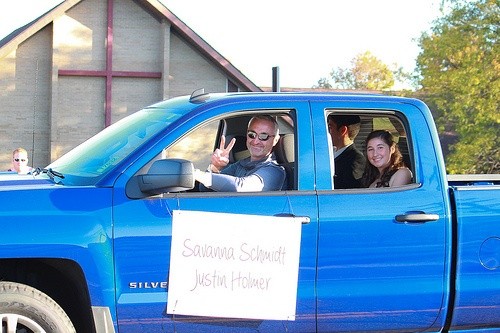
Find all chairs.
<box><xmin>223</xmin><ymin>134</ymin><xmax>295</xmax><ymax>190</ymax></box>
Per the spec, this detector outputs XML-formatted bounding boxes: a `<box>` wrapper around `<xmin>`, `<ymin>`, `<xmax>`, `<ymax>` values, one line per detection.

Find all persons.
<box><xmin>195</xmin><ymin>115</ymin><xmax>286</xmax><ymax>193</ymax></box>
<box><xmin>8</xmin><ymin>148</ymin><xmax>34</xmax><ymax>172</ymax></box>
<box><xmin>328</xmin><ymin>115</ymin><xmax>413</xmax><ymax>189</ymax></box>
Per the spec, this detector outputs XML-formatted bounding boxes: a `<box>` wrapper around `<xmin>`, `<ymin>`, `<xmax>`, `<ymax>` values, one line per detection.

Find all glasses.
<box><xmin>14</xmin><ymin>158</ymin><xmax>27</xmax><ymax>162</ymax></box>
<box><xmin>247</xmin><ymin>131</ymin><xmax>275</xmax><ymax>141</ymax></box>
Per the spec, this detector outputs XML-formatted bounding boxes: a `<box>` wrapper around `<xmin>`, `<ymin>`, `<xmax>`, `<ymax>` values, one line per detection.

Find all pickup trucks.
<box><xmin>0</xmin><ymin>88</ymin><xmax>500</xmax><ymax>333</ymax></box>
